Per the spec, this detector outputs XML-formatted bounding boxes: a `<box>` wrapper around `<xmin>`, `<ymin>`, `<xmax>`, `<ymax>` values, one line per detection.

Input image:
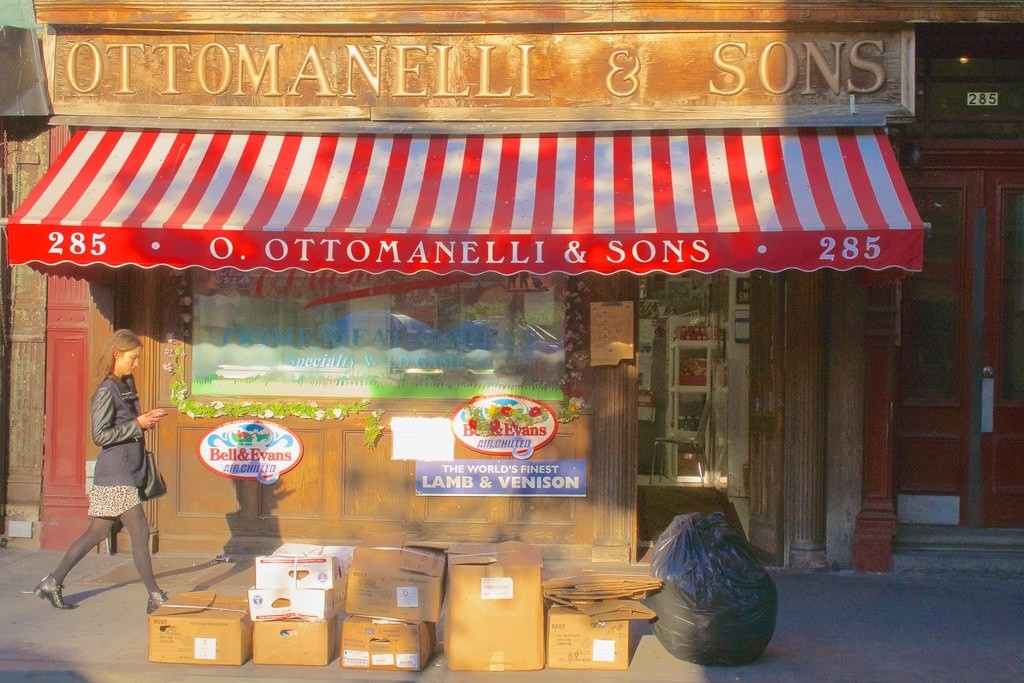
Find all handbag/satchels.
<box><xmin>139</xmin><ymin>450</ymin><xmax>166</xmax><ymax>500</ymax></box>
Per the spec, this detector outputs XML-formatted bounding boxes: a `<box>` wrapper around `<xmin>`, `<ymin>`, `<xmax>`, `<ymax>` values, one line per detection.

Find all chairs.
<box><xmin>650</xmin><ymin>402</ymin><xmax>715</xmax><ymax>488</ymax></box>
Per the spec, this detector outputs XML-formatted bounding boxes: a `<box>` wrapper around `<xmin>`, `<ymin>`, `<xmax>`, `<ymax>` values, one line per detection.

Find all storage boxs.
<box><xmin>148</xmin><ymin>590</ymin><xmax>253</xmax><ymax>666</ymax></box>
<box><xmin>246</xmin><ymin>542</ymin><xmax>355</xmax><ymax>665</ymax></box>
<box><xmin>345</xmin><ymin>541</ymin><xmax>448</xmax><ymax>624</ymax></box>
<box><xmin>547</xmin><ymin>601</ymin><xmax>630</xmax><ymax>670</ymax></box>
<box><xmin>342</xmin><ymin>614</ymin><xmax>437</xmax><ymax>673</ymax></box>
<box><xmin>445</xmin><ymin>540</ymin><xmax>547</xmax><ymax>672</ymax></box>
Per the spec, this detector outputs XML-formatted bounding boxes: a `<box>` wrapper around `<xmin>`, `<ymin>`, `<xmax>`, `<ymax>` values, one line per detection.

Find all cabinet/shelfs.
<box><xmin>667</xmin><ymin>311</ymin><xmax>715</xmax><ymax>485</ymax></box>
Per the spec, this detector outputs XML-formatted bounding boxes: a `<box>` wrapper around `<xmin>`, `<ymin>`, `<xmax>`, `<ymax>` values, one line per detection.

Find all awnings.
<box><xmin>8</xmin><ymin>126</ymin><xmax>928</xmax><ymax>276</ymax></box>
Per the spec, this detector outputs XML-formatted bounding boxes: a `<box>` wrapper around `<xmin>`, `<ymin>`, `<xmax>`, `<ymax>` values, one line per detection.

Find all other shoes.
<box><xmin>33</xmin><ymin>571</ymin><xmax>73</xmax><ymax>609</ymax></box>
<box><xmin>146</xmin><ymin>589</ymin><xmax>168</xmax><ymax>615</ymax></box>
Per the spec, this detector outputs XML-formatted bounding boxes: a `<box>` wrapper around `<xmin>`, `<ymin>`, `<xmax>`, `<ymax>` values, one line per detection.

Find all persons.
<box><xmin>34</xmin><ymin>329</ymin><xmax>169</xmax><ymax>614</ymax></box>
